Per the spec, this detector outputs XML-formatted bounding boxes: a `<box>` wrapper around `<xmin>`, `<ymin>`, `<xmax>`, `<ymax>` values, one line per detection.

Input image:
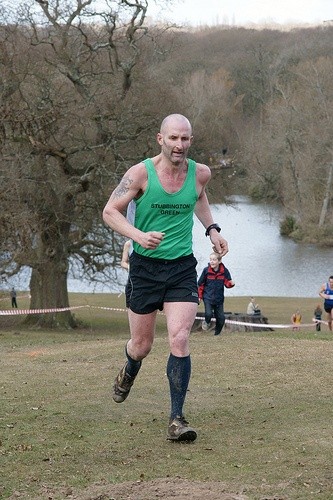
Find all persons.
<box><xmin>247</xmin><ymin>297</ymin><xmax>261</xmax><ymax>316</ymax></box>
<box><xmin>197</xmin><ymin>252</ymin><xmax>236</xmax><ymax>336</ymax></box>
<box><xmin>319</xmin><ymin>275</ymin><xmax>333</xmax><ymax>332</ymax></box>
<box><xmin>102</xmin><ymin>114</ymin><xmax>229</xmax><ymax>442</ymax></box>
<box><xmin>120</xmin><ymin>239</ymin><xmax>134</xmax><ymax>272</ymax></box>
<box><xmin>290</xmin><ymin>309</ymin><xmax>302</xmax><ymax>332</ymax></box>
<box><xmin>313</xmin><ymin>304</ymin><xmax>323</xmax><ymax>332</ymax></box>
<box><xmin>10</xmin><ymin>287</ymin><xmax>20</xmax><ymax>308</ymax></box>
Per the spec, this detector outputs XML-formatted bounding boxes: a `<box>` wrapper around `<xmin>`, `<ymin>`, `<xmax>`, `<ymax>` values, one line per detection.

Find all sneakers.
<box><xmin>113</xmin><ymin>361</ymin><xmax>141</xmax><ymax>404</ymax></box>
<box><xmin>166</xmin><ymin>418</ymin><xmax>197</xmax><ymax>442</ymax></box>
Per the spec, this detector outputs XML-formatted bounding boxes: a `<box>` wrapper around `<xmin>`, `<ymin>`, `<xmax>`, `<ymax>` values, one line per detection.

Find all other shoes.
<box><xmin>202</xmin><ymin>320</ymin><xmax>211</xmax><ymax>331</ymax></box>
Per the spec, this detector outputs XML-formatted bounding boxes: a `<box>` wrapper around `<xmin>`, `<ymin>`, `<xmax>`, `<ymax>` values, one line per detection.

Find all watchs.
<box><xmin>204</xmin><ymin>223</ymin><xmax>222</xmax><ymax>238</ymax></box>
